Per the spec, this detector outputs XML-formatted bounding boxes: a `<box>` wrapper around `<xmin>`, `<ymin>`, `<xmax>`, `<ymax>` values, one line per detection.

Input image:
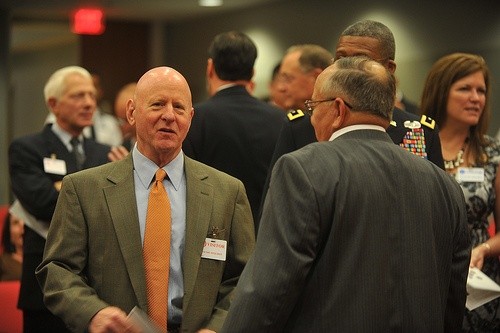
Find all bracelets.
<box><xmin>480</xmin><ymin>243</ymin><xmax>491</xmax><ymax>254</ymax></box>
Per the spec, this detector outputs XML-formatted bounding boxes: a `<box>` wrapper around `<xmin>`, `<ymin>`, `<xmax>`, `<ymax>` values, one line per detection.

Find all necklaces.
<box><xmin>443</xmin><ymin>137</ymin><xmax>470</xmax><ymax>170</ymax></box>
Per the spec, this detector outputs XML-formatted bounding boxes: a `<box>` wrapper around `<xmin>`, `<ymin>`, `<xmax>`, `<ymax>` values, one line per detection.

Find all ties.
<box><xmin>70</xmin><ymin>136</ymin><xmax>83</xmax><ymax>170</ymax></box>
<box><xmin>141</xmin><ymin>169</ymin><xmax>171</xmax><ymax>333</ymax></box>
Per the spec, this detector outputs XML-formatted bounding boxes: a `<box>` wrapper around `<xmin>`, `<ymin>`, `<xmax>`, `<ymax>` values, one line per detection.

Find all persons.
<box><xmin>416</xmin><ymin>52</ymin><xmax>500</xmax><ymax>333</ymax></box>
<box><xmin>7</xmin><ymin>65</ymin><xmax>128</xmax><ymax>333</ymax></box>
<box><xmin>34</xmin><ymin>66</ymin><xmax>255</xmax><ymax>333</ymax></box>
<box><xmin>0</xmin><ymin>213</ymin><xmax>25</xmax><ymax>333</ymax></box>
<box><xmin>219</xmin><ymin>55</ymin><xmax>471</xmax><ymax>333</ymax></box>
<box><xmin>42</xmin><ymin>19</ymin><xmax>440</xmax><ymax>236</ymax></box>
<box><xmin>182</xmin><ymin>29</ymin><xmax>286</xmax><ymax>219</ymax></box>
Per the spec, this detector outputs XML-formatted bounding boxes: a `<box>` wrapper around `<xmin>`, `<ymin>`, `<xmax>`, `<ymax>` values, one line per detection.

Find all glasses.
<box><xmin>304</xmin><ymin>99</ymin><xmax>355</xmax><ymax>115</ymax></box>
